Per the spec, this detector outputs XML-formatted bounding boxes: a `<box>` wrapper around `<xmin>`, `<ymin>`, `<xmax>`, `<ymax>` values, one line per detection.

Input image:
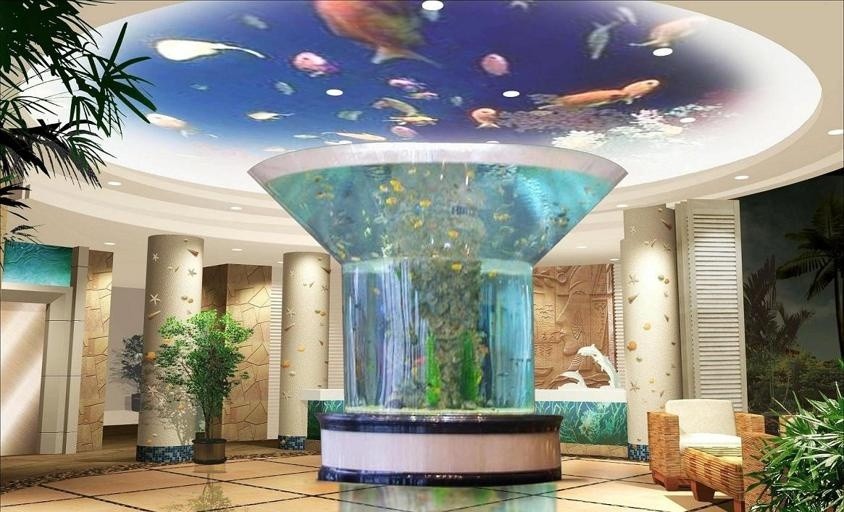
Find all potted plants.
<box><xmin>121</xmin><ymin>334</ymin><xmax>144</xmax><ymax>412</ymax></box>
<box><xmin>155</xmin><ymin>308</ymin><xmax>255</xmax><ymax>464</ymax></box>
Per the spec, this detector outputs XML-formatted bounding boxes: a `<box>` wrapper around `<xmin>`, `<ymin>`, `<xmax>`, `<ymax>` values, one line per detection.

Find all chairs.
<box><xmin>740</xmin><ymin>432</ymin><xmax>837</xmax><ymax>512</ymax></box>
<box><xmin>647</xmin><ymin>400</ymin><xmax>765</xmax><ymax>489</ymax></box>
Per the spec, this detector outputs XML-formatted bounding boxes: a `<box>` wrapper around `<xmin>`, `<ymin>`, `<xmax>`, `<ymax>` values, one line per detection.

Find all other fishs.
<box><xmin>305</xmin><ymin>156</ymin><xmax>597</xmax><ymax>297</ymax></box>
<box><xmin>143</xmin><ymin>1</ymin><xmax>702</xmax><ymax>145</ymax></box>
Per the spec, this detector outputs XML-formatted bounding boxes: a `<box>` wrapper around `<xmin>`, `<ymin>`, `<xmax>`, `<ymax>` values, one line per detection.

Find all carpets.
<box><xmin>665</xmin><ymin>496</ymin><xmax>747</xmax><ymax>512</ymax></box>
